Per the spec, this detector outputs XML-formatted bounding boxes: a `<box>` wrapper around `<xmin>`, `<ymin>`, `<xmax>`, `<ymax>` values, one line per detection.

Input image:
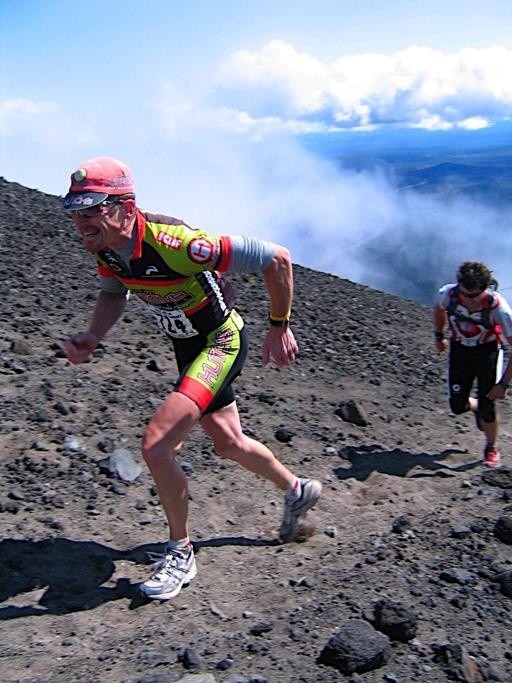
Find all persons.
<box><xmin>434</xmin><ymin>261</ymin><xmax>512</xmax><ymax>468</ymax></box>
<box><xmin>63</xmin><ymin>157</ymin><xmax>323</xmax><ymax>601</ymax></box>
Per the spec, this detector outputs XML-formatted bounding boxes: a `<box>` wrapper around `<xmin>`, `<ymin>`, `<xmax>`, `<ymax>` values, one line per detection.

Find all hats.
<box><xmin>60</xmin><ymin>155</ymin><xmax>137</xmax><ymax>213</ymax></box>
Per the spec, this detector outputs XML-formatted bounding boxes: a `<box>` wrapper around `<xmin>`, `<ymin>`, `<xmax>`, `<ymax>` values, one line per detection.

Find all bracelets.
<box><xmin>268</xmin><ymin>308</ymin><xmax>291</xmax><ymax>327</ymax></box>
<box><xmin>499</xmin><ymin>379</ymin><xmax>509</xmax><ymax>390</ymax></box>
<box><xmin>435</xmin><ymin>331</ymin><xmax>443</xmax><ymax>341</ymax></box>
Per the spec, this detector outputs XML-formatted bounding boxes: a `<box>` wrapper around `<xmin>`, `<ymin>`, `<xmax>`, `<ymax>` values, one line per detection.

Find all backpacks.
<box><xmin>448</xmin><ymin>274</ymin><xmax>498</xmax><ymax>330</ymax></box>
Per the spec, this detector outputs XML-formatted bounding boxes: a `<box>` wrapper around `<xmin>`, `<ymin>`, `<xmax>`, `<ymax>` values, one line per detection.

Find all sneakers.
<box><xmin>137</xmin><ymin>544</ymin><xmax>196</xmax><ymax>601</ymax></box>
<box><xmin>279</xmin><ymin>478</ymin><xmax>323</xmax><ymax>543</ymax></box>
<box><xmin>472</xmin><ymin>396</ymin><xmax>486</xmax><ymax>433</ymax></box>
<box><xmin>480</xmin><ymin>449</ymin><xmax>501</xmax><ymax>469</ymax></box>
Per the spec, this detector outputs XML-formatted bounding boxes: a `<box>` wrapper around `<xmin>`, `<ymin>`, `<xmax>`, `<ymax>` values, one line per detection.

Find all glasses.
<box><xmin>68</xmin><ymin>199</ymin><xmax>126</xmax><ymax>219</ymax></box>
<box><xmin>457</xmin><ymin>287</ymin><xmax>487</xmax><ymax>299</ymax></box>
